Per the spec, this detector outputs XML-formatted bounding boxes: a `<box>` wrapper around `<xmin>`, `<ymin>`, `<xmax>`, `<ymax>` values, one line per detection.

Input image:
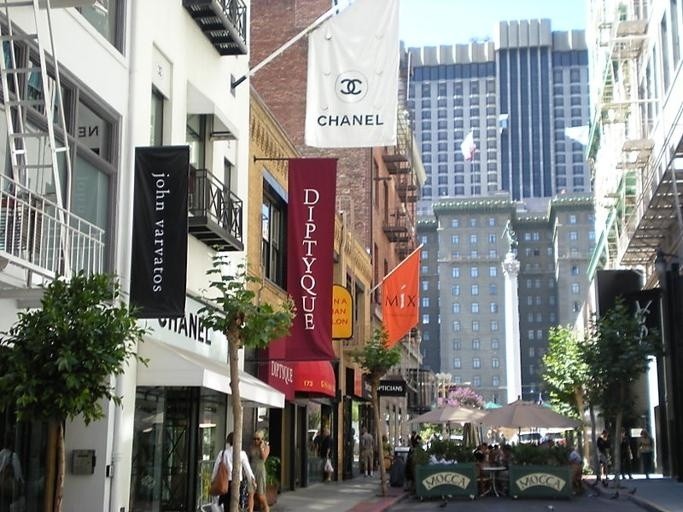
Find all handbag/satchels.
<box><xmin>324</xmin><ymin>458</ymin><xmax>334</xmax><ymax>472</ymax></box>
<box><xmin>208</xmin><ymin>464</ymin><xmax>228</xmax><ymax>496</ymax></box>
<box><xmin>240</xmin><ymin>481</ymin><xmax>248</xmax><ymax>509</ymax></box>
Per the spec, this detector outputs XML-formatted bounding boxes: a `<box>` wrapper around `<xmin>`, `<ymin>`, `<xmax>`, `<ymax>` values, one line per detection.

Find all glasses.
<box><xmin>253</xmin><ymin>437</ymin><xmax>260</xmax><ymax>441</ymax></box>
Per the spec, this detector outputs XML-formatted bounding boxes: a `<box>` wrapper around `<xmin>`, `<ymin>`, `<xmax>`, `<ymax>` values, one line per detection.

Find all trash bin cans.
<box><xmin>389</xmin><ymin>456</ymin><xmax>405</xmax><ymax>487</ymax></box>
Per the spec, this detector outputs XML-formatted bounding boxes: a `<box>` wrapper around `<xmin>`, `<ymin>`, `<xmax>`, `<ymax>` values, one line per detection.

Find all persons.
<box><xmin>411</xmin><ymin>426</ymin><xmax>581</xmax><ymax>465</ymax></box>
<box><xmin>310</xmin><ymin>431</ymin><xmax>320</xmax><ymax>455</ymax></box>
<box><xmin>360</xmin><ymin>427</ymin><xmax>392</xmax><ymax>476</ymax></box>
<box><xmin>211</xmin><ymin>428</ymin><xmax>270</xmax><ymax>512</ymax></box>
<box><xmin>597</xmin><ymin>427</ymin><xmax>654</xmax><ymax>479</ymax></box>
<box><xmin>320</xmin><ymin>429</ymin><xmax>334</xmax><ymax>481</ymax></box>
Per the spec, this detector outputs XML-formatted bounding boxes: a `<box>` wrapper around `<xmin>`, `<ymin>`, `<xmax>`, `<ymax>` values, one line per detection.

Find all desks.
<box><xmin>479</xmin><ymin>466</ymin><xmax>507</xmax><ymax>500</ymax></box>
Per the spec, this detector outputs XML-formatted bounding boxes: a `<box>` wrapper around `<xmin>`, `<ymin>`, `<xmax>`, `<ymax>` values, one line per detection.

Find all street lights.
<box><xmin>434</xmin><ymin>371</ymin><xmax>453</xmax><ymax>440</ymax></box>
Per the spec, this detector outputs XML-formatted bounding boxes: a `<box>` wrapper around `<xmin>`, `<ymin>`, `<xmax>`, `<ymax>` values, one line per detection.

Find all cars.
<box><xmin>542</xmin><ymin>432</ymin><xmax>565</xmax><ymax>447</ymax></box>
<box><xmin>516</xmin><ymin>431</ymin><xmax>545</xmax><ymax>448</ymax></box>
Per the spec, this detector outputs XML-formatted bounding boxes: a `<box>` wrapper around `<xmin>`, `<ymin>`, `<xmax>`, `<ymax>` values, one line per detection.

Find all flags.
<box><xmin>381</xmin><ymin>251</ymin><xmax>420</xmax><ymax>351</ymax></box>
<box><xmin>460</xmin><ymin>132</ymin><xmax>476</xmax><ymax>160</ymax></box>
<box><xmin>304</xmin><ymin>0</ymin><xmax>399</xmax><ymax>149</ymax></box>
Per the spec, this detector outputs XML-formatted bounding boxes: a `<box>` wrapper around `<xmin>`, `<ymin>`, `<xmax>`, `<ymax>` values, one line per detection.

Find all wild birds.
<box><xmin>545</xmin><ymin>503</ymin><xmax>555</xmax><ymax>511</ymax></box>
<box><xmin>610</xmin><ymin>489</ymin><xmax>620</xmax><ymax>500</ymax></box>
<box><xmin>629</xmin><ymin>486</ymin><xmax>637</xmax><ymax>494</ymax></box>
<box><xmin>435</xmin><ymin>499</ymin><xmax>449</xmax><ymax>509</ymax></box>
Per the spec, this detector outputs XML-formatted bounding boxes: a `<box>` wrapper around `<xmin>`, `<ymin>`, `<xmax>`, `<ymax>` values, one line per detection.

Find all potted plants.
<box><xmin>263</xmin><ymin>456</ymin><xmax>281</xmax><ymax>507</ymax></box>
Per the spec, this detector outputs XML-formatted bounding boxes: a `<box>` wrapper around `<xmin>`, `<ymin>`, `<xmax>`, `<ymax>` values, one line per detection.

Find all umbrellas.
<box><xmin>406</xmin><ymin>395</ymin><xmax>584</xmax><ymax>444</ymax></box>
